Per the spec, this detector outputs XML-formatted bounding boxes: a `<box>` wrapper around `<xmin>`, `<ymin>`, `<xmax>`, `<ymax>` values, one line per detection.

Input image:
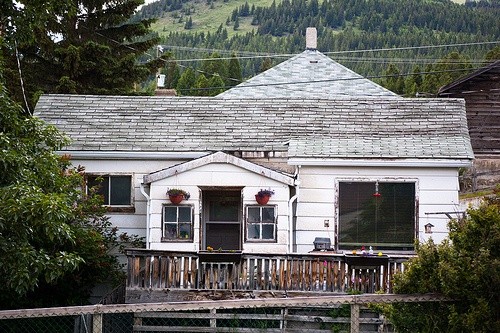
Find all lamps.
<box><xmin>424</xmin><ymin>214</ymin><xmax>435</xmax><ymax>234</ymax></box>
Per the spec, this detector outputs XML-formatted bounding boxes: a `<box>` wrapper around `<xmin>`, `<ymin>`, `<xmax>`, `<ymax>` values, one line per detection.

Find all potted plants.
<box><xmin>254</xmin><ymin>189</ymin><xmax>275</xmax><ymax>205</ymax></box>
<box><xmin>166</xmin><ymin>187</ymin><xmax>189</xmax><ymax>205</ymax></box>
